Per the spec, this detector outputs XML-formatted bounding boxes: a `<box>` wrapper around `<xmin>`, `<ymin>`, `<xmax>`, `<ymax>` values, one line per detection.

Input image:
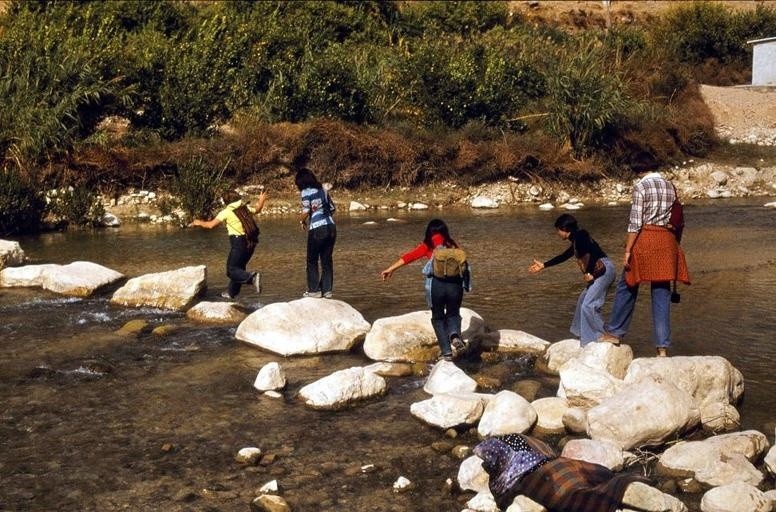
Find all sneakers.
<box><xmin>219</xmin><ymin>291</ymin><xmax>237</xmax><ymax>302</ymax></box>
<box><xmin>252</xmin><ymin>272</ymin><xmax>261</xmax><ymax>293</ymax></box>
<box><xmin>301</xmin><ymin>290</ymin><xmax>332</xmax><ymax>298</ymax></box>
<box><xmin>436</xmin><ymin>338</ymin><xmax>469</xmax><ymax>361</ymax></box>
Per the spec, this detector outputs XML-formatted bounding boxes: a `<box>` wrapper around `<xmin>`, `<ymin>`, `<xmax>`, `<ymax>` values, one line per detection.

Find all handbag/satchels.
<box><xmin>670</xmin><ymin>199</ymin><xmax>683</xmax><ymax>230</ymax></box>
<box><xmin>576</xmin><ymin>253</ymin><xmax>606</xmax><ymax>279</ymax></box>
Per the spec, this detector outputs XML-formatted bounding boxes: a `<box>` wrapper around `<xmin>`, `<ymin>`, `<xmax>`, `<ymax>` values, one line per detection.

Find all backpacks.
<box><xmin>432</xmin><ymin>247</ymin><xmax>467</xmax><ymax>279</ymax></box>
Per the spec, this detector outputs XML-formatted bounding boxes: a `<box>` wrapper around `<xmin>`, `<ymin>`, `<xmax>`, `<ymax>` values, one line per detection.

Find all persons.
<box><xmin>381</xmin><ymin>219</ymin><xmax>472</xmax><ymax>361</ymax></box>
<box><xmin>185</xmin><ymin>189</ymin><xmax>272</xmax><ymax>299</ymax></box>
<box><xmin>293</xmin><ymin>168</ymin><xmax>338</xmax><ymax>299</ymax></box>
<box><xmin>529</xmin><ymin>214</ymin><xmax>617</xmax><ymax>347</ymax></box>
<box><xmin>595</xmin><ymin>148</ymin><xmax>692</xmax><ymax>357</ymax></box>
